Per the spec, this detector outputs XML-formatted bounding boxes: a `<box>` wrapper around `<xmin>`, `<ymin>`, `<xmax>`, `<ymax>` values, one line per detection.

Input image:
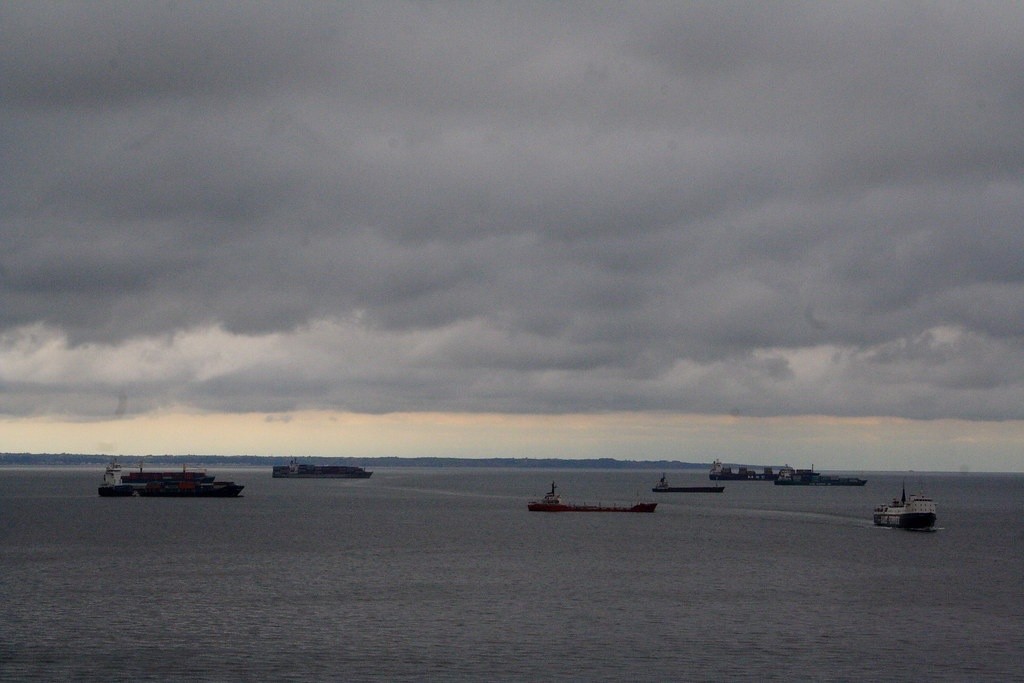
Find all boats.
<box><xmin>98</xmin><ymin>457</ymin><xmax>245</xmax><ymax>498</ymax></box>
<box><xmin>774</xmin><ymin>462</ymin><xmax>868</xmax><ymax>487</ymax></box>
<box><xmin>526</xmin><ymin>480</ymin><xmax>657</xmax><ymax>513</ymax></box>
<box><xmin>651</xmin><ymin>472</ymin><xmax>725</xmax><ymax>492</ymax></box>
<box><xmin>271</xmin><ymin>464</ymin><xmax>374</xmax><ymax>479</ymax></box>
<box><xmin>709</xmin><ymin>456</ymin><xmax>780</xmax><ymax>480</ymax></box>
<box><xmin>874</xmin><ymin>482</ymin><xmax>937</xmax><ymax>529</ymax></box>
